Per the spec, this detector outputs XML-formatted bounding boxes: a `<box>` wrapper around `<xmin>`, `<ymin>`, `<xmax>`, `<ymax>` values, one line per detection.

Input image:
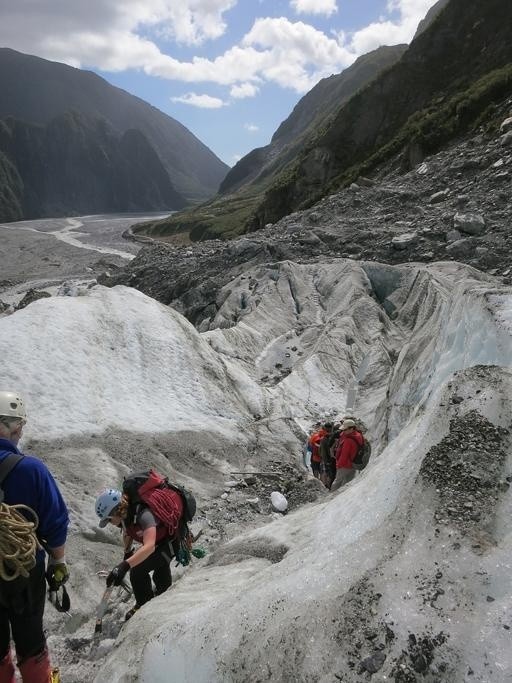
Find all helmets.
<box><xmin>95</xmin><ymin>489</ymin><xmax>122</xmax><ymax>528</ymax></box>
<box><xmin>0</xmin><ymin>392</ymin><xmax>27</xmax><ymax>421</ymax></box>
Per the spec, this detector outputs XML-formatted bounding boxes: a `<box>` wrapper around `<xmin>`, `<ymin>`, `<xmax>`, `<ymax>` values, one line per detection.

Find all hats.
<box><xmin>322</xmin><ymin>422</ymin><xmax>331</xmax><ymax>432</ymax></box>
<box><xmin>339</xmin><ymin>419</ymin><xmax>355</xmax><ymax>430</ymax></box>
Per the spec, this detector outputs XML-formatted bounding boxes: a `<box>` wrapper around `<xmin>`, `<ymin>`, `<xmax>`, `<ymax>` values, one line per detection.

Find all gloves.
<box><xmin>123</xmin><ymin>548</ymin><xmax>135</xmax><ymax>560</ymax></box>
<box><xmin>47</xmin><ymin>555</ymin><xmax>69</xmax><ymax>588</ymax></box>
<box><xmin>106</xmin><ymin>561</ymin><xmax>131</xmax><ymax>587</ymax></box>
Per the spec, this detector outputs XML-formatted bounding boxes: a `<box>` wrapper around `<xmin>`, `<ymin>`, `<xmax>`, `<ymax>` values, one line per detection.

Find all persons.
<box><xmin>327</xmin><ymin>420</ymin><xmax>345</xmax><ymax>488</ymax></box>
<box><xmin>306</xmin><ymin>423</ymin><xmax>327</xmax><ymax>479</ymax></box>
<box><xmin>80</xmin><ymin>487</ymin><xmax>190</xmax><ymax>622</ymax></box>
<box><xmin>317</xmin><ymin>420</ymin><xmax>333</xmax><ymax>488</ymax></box>
<box><xmin>329</xmin><ymin>417</ymin><xmax>365</xmax><ymax>493</ymax></box>
<box><xmin>0</xmin><ymin>388</ymin><xmax>71</xmax><ymax>682</ymax></box>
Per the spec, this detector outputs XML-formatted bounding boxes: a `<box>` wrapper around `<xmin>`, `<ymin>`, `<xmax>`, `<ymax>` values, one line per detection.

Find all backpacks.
<box><xmin>346</xmin><ymin>430</ymin><xmax>371</xmax><ymax>470</ymax></box>
<box><xmin>123</xmin><ymin>469</ymin><xmax>196</xmax><ymax>525</ymax></box>
